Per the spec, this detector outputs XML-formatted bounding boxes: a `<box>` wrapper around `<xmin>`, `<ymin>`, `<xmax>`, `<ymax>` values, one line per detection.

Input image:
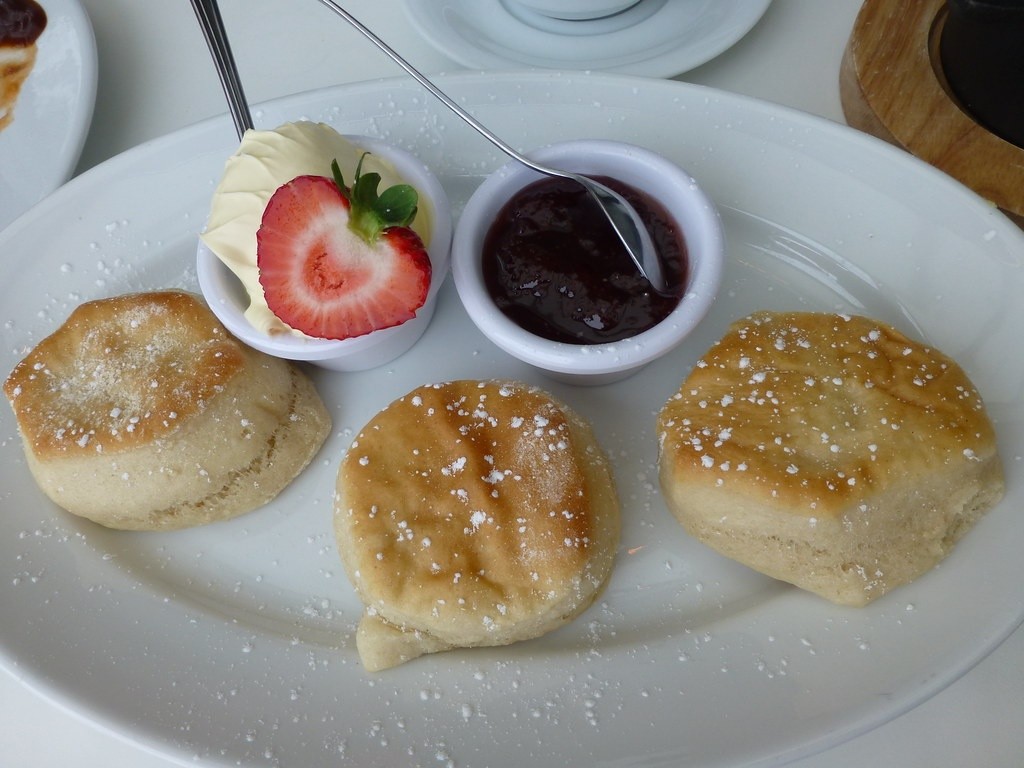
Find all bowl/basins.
<box><xmin>195</xmin><ymin>136</ymin><xmax>451</xmax><ymax>371</ymax></box>
<box><xmin>451</xmin><ymin>140</ymin><xmax>725</xmax><ymax>387</ymax></box>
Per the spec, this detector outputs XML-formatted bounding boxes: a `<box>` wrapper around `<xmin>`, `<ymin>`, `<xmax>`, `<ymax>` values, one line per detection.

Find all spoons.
<box><xmin>320</xmin><ymin>0</ymin><xmax>665</xmax><ymax>292</ymax></box>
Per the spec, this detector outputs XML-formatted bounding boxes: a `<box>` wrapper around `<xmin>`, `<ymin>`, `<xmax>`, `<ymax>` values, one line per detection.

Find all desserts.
<box><xmin>204</xmin><ymin>121</ymin><xmax>431</xmax><ymax>334</ymax></box>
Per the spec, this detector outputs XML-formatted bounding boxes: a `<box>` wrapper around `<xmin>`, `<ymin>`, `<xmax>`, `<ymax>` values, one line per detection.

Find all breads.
<box><xmin>2</xmin><ymin>289</ymin><xmax>332</xmax><ymax>532</ymax></box>
<box><xmin>337</xmin><ymin>381</ymin><xmax>623</xmax><ymax>673</ymax></box>
<box><xmin>659</xmin><ymin>315</ymin><xmax>1010</xmax><ymax>604</ymax></box>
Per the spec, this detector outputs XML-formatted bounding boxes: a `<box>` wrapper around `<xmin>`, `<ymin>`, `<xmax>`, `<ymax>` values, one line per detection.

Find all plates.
<box><xmin>0</xmin><ymin>70</ymin><xmax>1024</xmax><ymax>768</ymax></box>
<box><xmin>0</xmin><ymin>0</ymin><xmax>98</xmax><ymax>235</ymax></box>
<box><xmin>400</xmin><ymin>1</ymin><xmax>771</xmax><ymax>79</ymax></box>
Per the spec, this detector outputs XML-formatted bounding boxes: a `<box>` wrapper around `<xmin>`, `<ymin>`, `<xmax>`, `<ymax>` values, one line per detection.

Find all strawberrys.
<box><xmin>256</xmin><ymin>176</ymin><xmax>432</xmax><ymax>340</ymax></box>
